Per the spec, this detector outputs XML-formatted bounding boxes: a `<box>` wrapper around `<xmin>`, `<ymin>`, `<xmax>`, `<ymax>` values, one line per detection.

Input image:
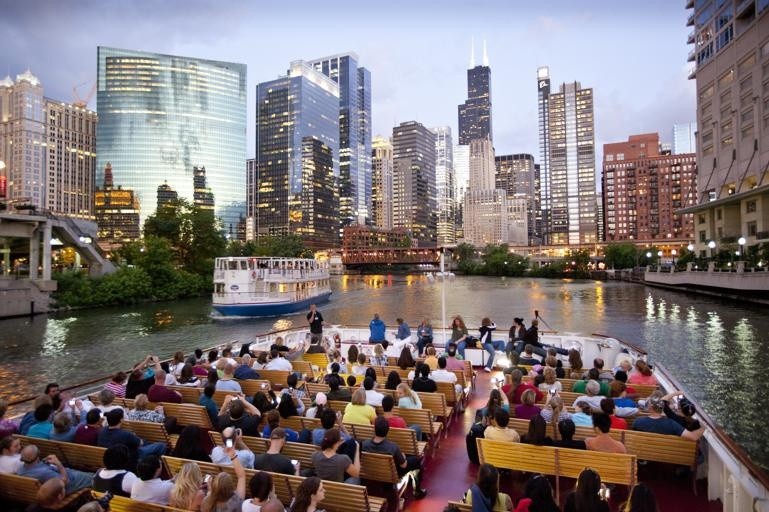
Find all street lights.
<box><xmin>658</xmin><ymin>250</ymin><xmax>663</xmax><ymax>265</ymax></box>
<box><xmin>671</xmin><ymin>250</ymin><xmax>676</xmax><ymax>265</ymax></box>
<box><xmin>687</xmin><ymin>244</ymin><xmax>693</xmax><ymax>261</ymax></box>
<box><xmin>426</xmin><ymin>252</ymin><xmax>456</xmax><ymax>346</ymax></box>
<box><xmin>708</xmin><ymin>240</ymin><xmax>717</xmax><ymax>261</ymax></box>
<box><xmin>737</xmin><ymin>237</ymin><xmax>746</xmax><ymax>261</ymax></box>
<box><xmin>646</xmin><ymin>252</ymin><xmax>652</xmax><ymax>265</ymax></box>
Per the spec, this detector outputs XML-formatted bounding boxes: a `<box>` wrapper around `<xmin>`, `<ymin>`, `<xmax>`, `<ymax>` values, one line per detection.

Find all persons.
<box><xmin>1</xmin><ymin>304</ymin><xmax>710</xmax><ymax>512</ymax></box>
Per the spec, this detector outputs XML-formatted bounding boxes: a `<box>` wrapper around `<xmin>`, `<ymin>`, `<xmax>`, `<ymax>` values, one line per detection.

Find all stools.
<box><xmin>422</xmin><ymin>347</ymin><xmax>542</xmax><ymax>367</ymax></box>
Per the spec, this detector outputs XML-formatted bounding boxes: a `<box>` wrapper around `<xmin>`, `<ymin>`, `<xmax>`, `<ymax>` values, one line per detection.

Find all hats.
<box><xmin>650</xmin><ymin>395</ymin><xmax>666</xmax><ymax>415</ymax></box>
<box><xmin>267</xmin><ymin>427</ymin><xmax>286</xmax><ymax>442</ymax></box>
<box><xmin>679</xmin><ymin>397</ymin><xmax>697</xmax><ymax>418</ymax></box>
<box><xmin>315</xmin><ymin>390</ymin><xmax>328</xmax><ymax>407</ymax></box>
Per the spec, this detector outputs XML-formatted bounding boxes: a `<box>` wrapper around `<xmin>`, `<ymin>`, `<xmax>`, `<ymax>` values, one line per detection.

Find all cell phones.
<box><xmin>68</xmin><ymin>400</ymin><xmax>76</xmax><ymax>405</ymax></box>
<box><xmin>550</xmin><ymin>389</ymin><xmax>556</xmax><ymax>394</ymax></box>
<box><xmin>291</xmin><ymin>460</ymin><xmax>299</xmax><ymax>465</ymax></box>
<box><xmin>678</xmin><ymin>395</ymin><xmax>684</xmax><ymax>402</ymax></box>
<box><xmin>203</xmin><ymin>473</ymin><xmax>212</xmax><ymax>483</ymax></box>
<box><xmin>342</xmin><ymin>362</ymin><xmax>344</xmax><ymax>365</ymax></box>
<box><xmin>170</xmin><ymin>367</ymin><xmax>173</xmax><ymax>372</ymax></box>
<box><xmin>150</xmin><ymin>357</ymin><xmax>153</xmax><ymax>361</ymax></box>
<box><xmin>289</xmin><ymin>387</ymin><xmax>293</xmax><ymax>393</ymax></box>
<box><xmin>231</xmin><ymin>397</ymin><xmax>238</xmax><ymax>402</ymax></box>
<box><xmin>261</xmin><ymin>384</ymin><xmax>268</xmax><ymax>388</ymax></box>
<box><xmin>226</xmin><ymin>438</ymin><xmax>233</xmax><ymax>449</ymax></box>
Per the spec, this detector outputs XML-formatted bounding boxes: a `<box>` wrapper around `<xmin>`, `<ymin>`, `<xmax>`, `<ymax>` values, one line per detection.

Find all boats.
<box><xmin>212</xmin><ymin>255</ymin><xmax>333</xmax><ymax>316</ymax></box>
<box><xmin>329</xmin><ymin>253</ymin><xmax>347</xmax><ymax>274</ymax></box>
<box><xmin>0</xmin><ymin>323</ymin><xmax>769</xmax><ymax>511</ymax></box>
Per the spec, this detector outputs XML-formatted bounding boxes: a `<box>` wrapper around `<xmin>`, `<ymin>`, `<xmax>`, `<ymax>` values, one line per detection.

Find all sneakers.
<box><xmin>482</xmin><ymin>365</ymin><xmax>491</xmax><ymax>374</ymax></box>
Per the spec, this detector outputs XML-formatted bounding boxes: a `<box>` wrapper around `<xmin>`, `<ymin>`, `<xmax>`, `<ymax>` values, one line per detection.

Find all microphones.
<box><xmin>314</xmin><ymin>311</ymin><xmax>316</xmax><ymax>314</ymax></box>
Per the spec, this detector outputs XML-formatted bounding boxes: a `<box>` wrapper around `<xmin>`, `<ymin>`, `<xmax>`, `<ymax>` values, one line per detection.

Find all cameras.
<box><xmin>96</xmin><ymin>490</ymin><xmax>113</xmax><ymax>508</ymax></box>
